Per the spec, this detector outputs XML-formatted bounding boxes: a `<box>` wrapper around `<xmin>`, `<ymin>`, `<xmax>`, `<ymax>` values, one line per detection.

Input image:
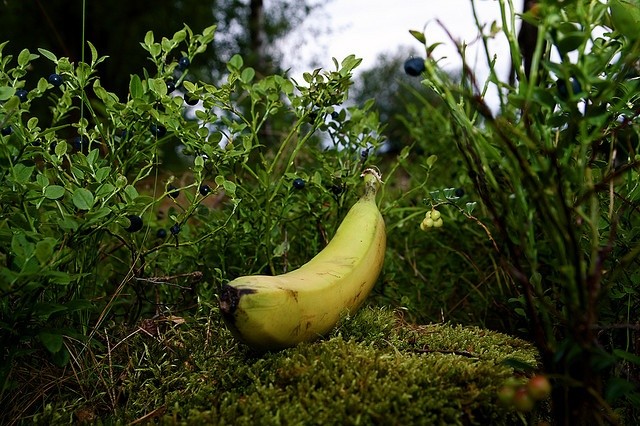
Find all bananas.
<box><xmin>219</xmin><ymin>170</ymin><xmax>386</xmax><ymax>354</ymax></box>
